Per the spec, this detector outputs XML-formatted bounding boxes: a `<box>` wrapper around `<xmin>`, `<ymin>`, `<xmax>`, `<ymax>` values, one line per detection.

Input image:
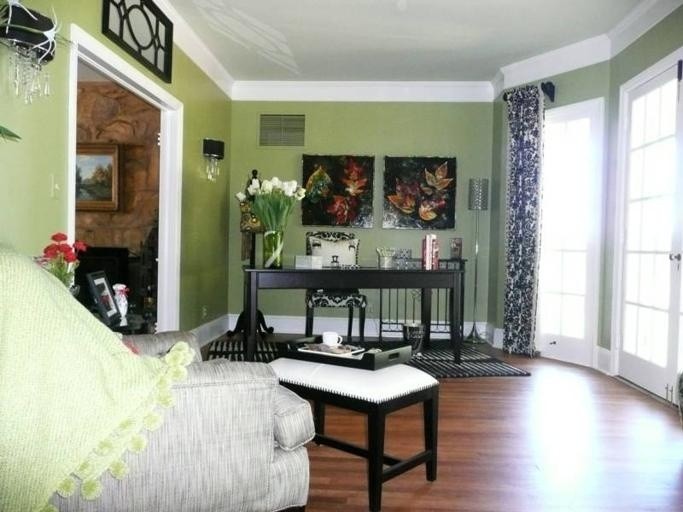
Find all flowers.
<box><xmin>236</xmin><ymin>176</ymin><xmax>307</xmax><ymax>231</ymax></box>
<box><xmin>38</xmin><ymin>232</ymin><xmax>88</xmax><ymax>288</ymax></box>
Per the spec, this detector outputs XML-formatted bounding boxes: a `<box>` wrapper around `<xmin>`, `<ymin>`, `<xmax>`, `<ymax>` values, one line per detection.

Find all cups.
<box><xmin>323</xmin><ymin>331</ymin><xmax>343</xmax><ymax>347</ymax></box>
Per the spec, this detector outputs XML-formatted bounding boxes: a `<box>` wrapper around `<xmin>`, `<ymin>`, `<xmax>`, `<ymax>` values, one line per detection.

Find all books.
<box><xmin>421</xmin><ymin>233</ymin><xmax>439</xmax><ymax>271</ymax></box>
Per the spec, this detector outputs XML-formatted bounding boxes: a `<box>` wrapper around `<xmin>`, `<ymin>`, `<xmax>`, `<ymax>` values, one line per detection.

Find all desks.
<box><xmin>244</xmin><ymin>268</ymin><xmax>465</xmax><ymax>362</ymax></box>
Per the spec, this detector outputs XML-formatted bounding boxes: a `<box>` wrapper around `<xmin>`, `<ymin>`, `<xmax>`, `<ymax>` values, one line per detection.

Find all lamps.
<box><xmin>203</xmin><ymin>139</ymin><xmax>225</xmax><ymax>181</ymax></box>
<box><xmin>468</xmin><ymin>178</ymin><xmax>489</xmax><ymax>343</ymax></box>
<box><xmin>0</xmin><ymin>0</ymin><xmax>58</xmax><ymax>105</ymax></box>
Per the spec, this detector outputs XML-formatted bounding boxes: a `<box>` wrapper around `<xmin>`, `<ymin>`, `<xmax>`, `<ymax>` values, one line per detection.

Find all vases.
<box><xmin>262</xmin><ymin>229</ymin><xmax>282</xmax><ymax>269</ymax></box>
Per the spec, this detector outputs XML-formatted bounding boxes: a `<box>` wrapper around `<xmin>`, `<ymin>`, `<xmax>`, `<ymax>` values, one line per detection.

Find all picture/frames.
<box><xmin>86</xmin><ymin>270</ymin><xmax>121</xmax><ymax>326</ymax></box>
<box><xmin>76</xmin><ymin>142</ymin><xmax>124</xmax><ymax>213</ymax></box>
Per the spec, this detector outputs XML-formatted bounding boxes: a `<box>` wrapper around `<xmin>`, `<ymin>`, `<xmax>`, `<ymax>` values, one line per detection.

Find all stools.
<box><xmin>269</xmin><ymin>357</ymin><xmax>440</xmax><ymax>512</ymax></box>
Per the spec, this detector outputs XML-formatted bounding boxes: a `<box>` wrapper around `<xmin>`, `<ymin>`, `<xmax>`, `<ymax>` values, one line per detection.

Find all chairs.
<box><xmin>0</xmin><ymin>254</ymin><xmax>316</xmax><ymax>512</ymax></box>
<box><xmin>306</xmin><ymin>231</ymin><xmax>366</xmax><ymax>344</ymax></box>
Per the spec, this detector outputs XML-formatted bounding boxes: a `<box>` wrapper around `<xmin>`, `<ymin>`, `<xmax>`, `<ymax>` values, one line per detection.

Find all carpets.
<box><xmin>207</xmin><ymin>339</ymin><xmax>531</xmax><ymax>377</ymax></box>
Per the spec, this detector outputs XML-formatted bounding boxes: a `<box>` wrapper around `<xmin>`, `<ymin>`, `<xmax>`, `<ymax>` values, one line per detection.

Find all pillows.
<box><xmin>311</xmin><ymin>237</ymin><xmax>359</xmax><ymax>266</ymax></box>
